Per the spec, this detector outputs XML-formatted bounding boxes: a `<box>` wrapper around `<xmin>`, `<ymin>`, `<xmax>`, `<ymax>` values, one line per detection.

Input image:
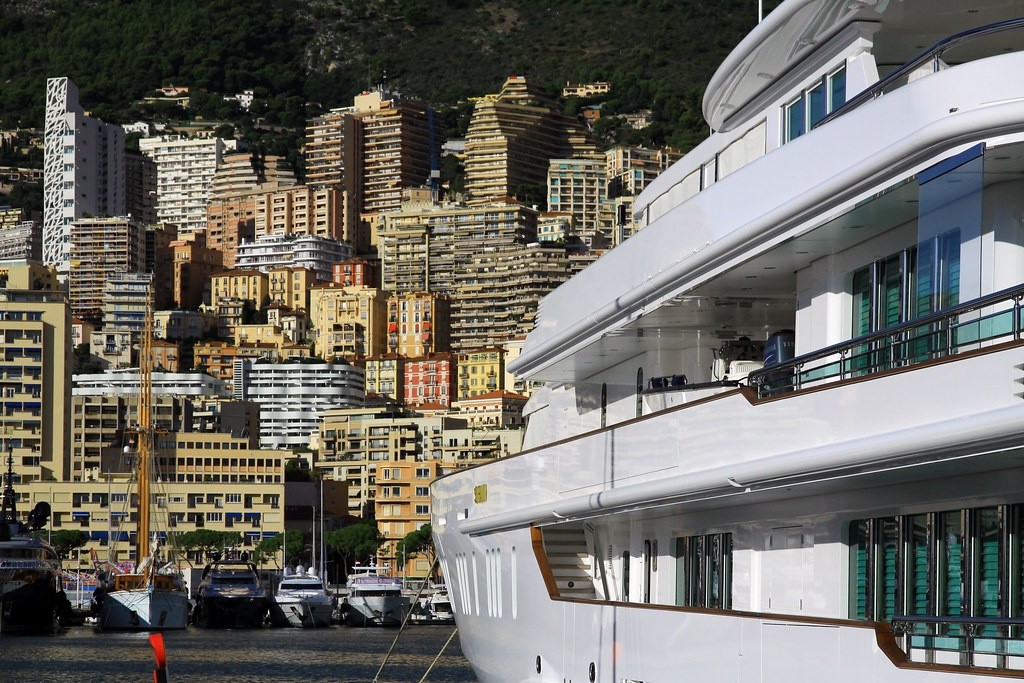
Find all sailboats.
<box><xmin>93</xmin><ymin>277</ymin><xmax>191</xmax><ymax>627</ymax></box>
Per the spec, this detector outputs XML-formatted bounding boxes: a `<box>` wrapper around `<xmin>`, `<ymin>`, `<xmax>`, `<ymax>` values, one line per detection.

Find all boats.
<box><xmin>409</xmin><ymin>580</ymin><xmax>457</xmax><ymax>626</ymax></box>
<box><xmin>271</xmin><ymin>565</ymin><xmax>334</xmax><ymax>630</ymax></box>
<box><xmin>0</xmin><ymin>447</ymin><xmax>72</xmax><ymax>636</ymax></box>
<box><xmin>194</xmin><ymin>549</ymin><xmax>269</xmax><ymax>627</ymax></box>
<box><xmin>428</xmin><ymin>0</ymin><xmax>1024</xmax><ymax>682</ymax></box>
<box><xmin>336</xmin><ymin>553</ymin><xmax>408</xmax><ymax>627</ymax></box>
